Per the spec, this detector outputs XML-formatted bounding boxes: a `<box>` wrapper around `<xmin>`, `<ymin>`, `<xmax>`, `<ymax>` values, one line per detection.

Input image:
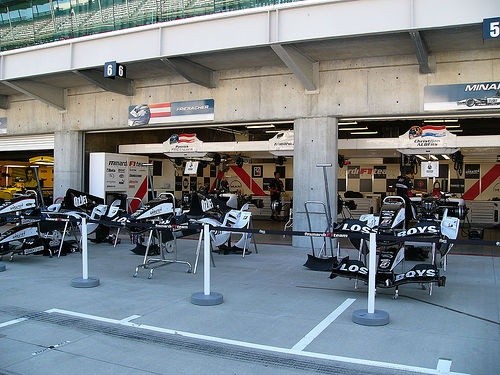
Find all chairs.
<box><xmin>218</xmin><ymin>210</ymin><xmax>258</xmax><ymax>258</ymax></box>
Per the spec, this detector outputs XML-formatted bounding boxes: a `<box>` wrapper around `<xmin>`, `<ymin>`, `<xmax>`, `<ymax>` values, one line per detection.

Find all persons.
<box><xmin>269</xmin><ymin>171</ymin><xmax>285</xmax><ymax>222</ymax></box>
<box><xmin>221</xmin><ymin>177</ymin><xmax>229</xmax><ymax>193</ymax></box>
<box><xmin>23</xmin><ymin>168</ymin><xmax>37</xmax><ymax>187</ymax></box>
<box><xmin>395</xmin><ymin>168</ymin><xmax>414</xmax><ymax>230</ymax></box>
<box><xmin>191</xmin><ymin>183</ymin><xmax>210</xmax><ymax>215</ymax></box>
